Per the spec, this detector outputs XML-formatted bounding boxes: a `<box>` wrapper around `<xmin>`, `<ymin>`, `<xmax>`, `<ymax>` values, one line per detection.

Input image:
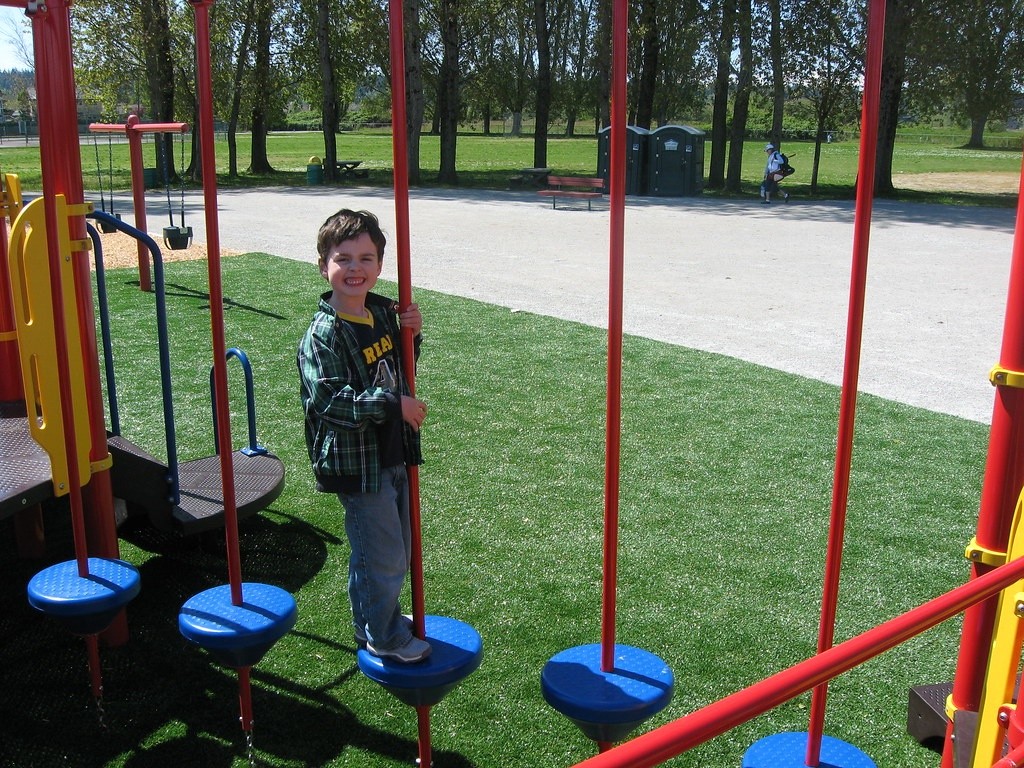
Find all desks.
<box><xmin>334</xmin><ymin>160</ymin><xmax>363</xmax><ymax>177</ymax></box>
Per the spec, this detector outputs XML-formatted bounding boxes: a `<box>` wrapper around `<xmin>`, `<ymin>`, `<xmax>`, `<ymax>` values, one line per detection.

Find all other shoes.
<box><xmin>761</xmin><ymin>201</ymin><xmax>770</xmax><ymax>204</ymax></box>
<box><xmin>785</xmin><ymin>194</ymin><xmax>790</xmax><ymax>203</ymax></box>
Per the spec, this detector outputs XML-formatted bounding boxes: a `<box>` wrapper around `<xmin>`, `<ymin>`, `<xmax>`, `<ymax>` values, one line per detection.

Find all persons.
<box><xmin>295</xmin><ymin>206</ymin><xmax>435</xmax><ymax>665</ymax></box>
<box><xmin>827</xmin><ymin>132</ymin><xmax>832</xmax><ymax>144</ymax></box>
<box><xmin>760</xmin><ymin>144</ymin><xmax>790</xmax><ymax>204</ymax></box>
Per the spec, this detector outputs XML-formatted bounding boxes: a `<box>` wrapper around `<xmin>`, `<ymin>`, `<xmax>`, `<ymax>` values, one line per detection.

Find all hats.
<box><xmin>765</xmin><ymin>144</ymin><xmax>774</xmax><ymax>151</ymax></box>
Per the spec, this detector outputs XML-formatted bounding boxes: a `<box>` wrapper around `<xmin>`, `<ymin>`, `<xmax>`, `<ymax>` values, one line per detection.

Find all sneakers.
<box><xmin>354</xmin><ymin>615</ymin><xmax>432</xmax><ymax>662</ymax></box>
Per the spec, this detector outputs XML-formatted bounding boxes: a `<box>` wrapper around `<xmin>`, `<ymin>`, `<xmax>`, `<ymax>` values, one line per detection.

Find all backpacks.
<box><xmin>774</xmin><ymin>153</ymin><xmax>795</xmax><ymax>176</ymax></box>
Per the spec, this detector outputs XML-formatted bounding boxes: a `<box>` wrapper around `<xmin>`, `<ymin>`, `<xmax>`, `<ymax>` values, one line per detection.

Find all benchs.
<box><xmin>536</xmin><ymin>175</ymin><xmax>605</xmax><ymax>210</ymax></box>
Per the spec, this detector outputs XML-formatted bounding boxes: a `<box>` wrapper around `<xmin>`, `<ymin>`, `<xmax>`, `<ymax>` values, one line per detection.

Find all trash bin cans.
<box><xmin>142</xmin><ymin>167</ymin><xmax>157</xmax><ymax>189</ymax></box>
<box><xmin>594</xmin><ymin>124</ymin><xmax>653</xmax><ymax>196</ymax></box>
<box><xmin>306</xmin><ymin>162</ymin><xmax>324</xmax><ymax>186</ymax></box>
<box><xmin>646</xmin><ymin>124</ymin><xmax>707</xmax><ymax>198</ymax></box>
